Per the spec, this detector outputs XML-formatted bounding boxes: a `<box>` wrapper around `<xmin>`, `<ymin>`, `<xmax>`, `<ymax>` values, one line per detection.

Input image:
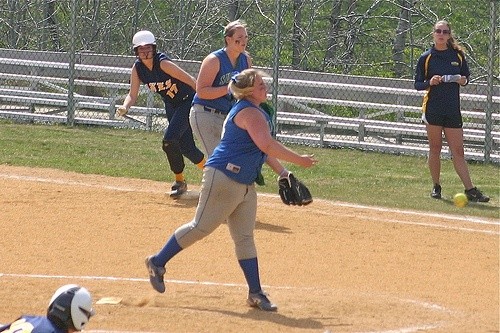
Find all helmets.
<box><xmin>132</xmin><ymin>31</ymin><xmax>158</xmax><ymax>56</ymax></box>
<box><xmin>47</xmin><ymin>284</ymin><xmax>95</xmax><ymax>331</ymax></box>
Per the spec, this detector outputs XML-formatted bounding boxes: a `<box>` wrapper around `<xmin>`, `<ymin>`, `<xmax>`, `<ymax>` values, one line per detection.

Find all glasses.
<box><xmin>434</xmin><ymin>29</ymin><xmax>450</xmax><ymax>34</ymax></box>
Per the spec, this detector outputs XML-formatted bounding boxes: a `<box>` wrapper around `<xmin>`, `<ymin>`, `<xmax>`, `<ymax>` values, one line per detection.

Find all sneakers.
<box><xmin>145</xmin><ymin>254</ymin><xmax>166</xmax><ymax>293</ymax></box>
<box><xmin>169</xmin><ymin>180</ymin><xmax>188</xmax><ymax>198</ymax></box>
<box><xmin>464</xmin><ymin>187</ymin><xmax>489</xmax><ymax>202</ymax></box>
<box><xmin>246</xmin><ymin>290</ymin><xmax>277</xmax><ymax>311</ymax></box>
<box><xmin>430</xmin><ymin>183</ymin><xmax>441</xmax><ymax>198</ymax></box>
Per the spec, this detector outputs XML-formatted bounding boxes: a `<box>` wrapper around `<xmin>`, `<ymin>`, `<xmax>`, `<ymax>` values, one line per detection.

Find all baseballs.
<box><xmin>454</xmin><ymin>193</ymin><xmax>468</xmax><ymax>208</ymax></box>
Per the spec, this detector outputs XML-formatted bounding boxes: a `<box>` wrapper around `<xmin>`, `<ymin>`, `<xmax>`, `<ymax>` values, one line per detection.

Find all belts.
<box><xmin>204</xmin><ymin>106</ymin><xmax>225</xmax><ymax>114</ymax></box>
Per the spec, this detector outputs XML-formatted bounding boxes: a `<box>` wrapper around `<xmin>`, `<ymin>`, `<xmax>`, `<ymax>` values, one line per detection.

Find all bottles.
<box><xmin>439</xmin><ymin>74</ymin><xmax>461</xmax><ymax>83</ymax></box>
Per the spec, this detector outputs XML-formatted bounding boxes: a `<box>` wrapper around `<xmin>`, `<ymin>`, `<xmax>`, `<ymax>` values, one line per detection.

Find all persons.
<box><xmin>146</xmin><ymin>68</ymin><xmax>318</xmax><ymax>312</ymax></box>
<box><xmin>0</xmin><ymin>284</ymin><xmax>95</xmax><ymax>333</ymax></box>
<box><xmin>415</xmin><ymin>22</ymin><xmax>490</xmax><ymax>202</ymax></box>
<box><xmin>188</xmin><ymin>21</ymin><xmax>253</xmax><ymax>162</ymax></box>
<box><xmin>117</xmin><ymin>31</ymin><xmax>208</xmax><ymax>198</ymax></box>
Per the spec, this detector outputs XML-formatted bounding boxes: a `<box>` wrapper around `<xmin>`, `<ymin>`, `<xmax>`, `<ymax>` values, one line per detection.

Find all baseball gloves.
<box><xmin>276</xmin><ymin>172</ymin><xmax>313</xmax><ymax>207</ymax></box>
